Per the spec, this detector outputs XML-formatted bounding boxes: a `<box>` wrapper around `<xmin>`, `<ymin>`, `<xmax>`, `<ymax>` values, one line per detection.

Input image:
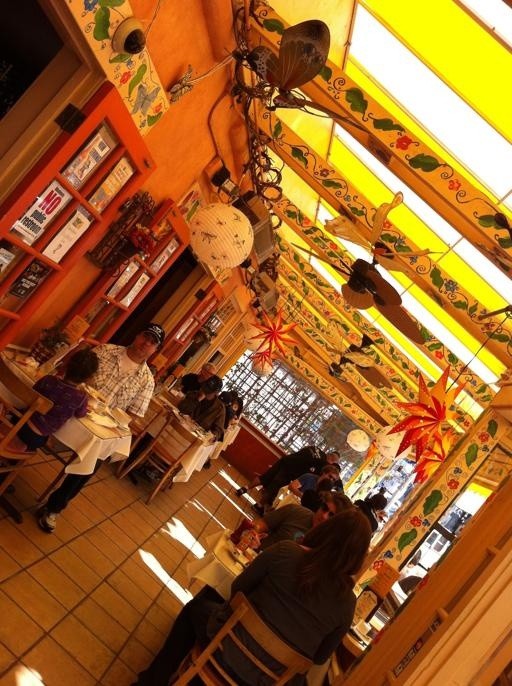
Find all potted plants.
<box><xmin>29</xmin><ymin>321</ymin><xmax>70</xmax><ymax>363</ymax></box>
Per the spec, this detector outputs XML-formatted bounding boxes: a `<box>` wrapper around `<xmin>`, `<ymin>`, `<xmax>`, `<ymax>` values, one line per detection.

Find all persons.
<box><xmin>180</xmin><ymin>361</ymin><xmax>217</xmax><ymax>394</ymax></box>
<box><xmin>238</xmin><ymin>444</ymin><xmax>340</xmax><ymax>512</ymax></box>
<box><xmin>378</xmin><ymin>486</ymin><xmax>388</xmax><ymax>495</ymax></box>
<box><xmin>301</xmin><ymin>479</ymin><xmax>333</xmax><ymax>512</ymax></box>
<box><xmin>251</xmin><ymin>492</ymin><xmax>355</xmax><ymax>556</ymax></box>
<box><xmin>354</xmin><ymin>493</ymin><xmax>387</xmax><ymax>534</ymax></box>
<box><xmin>288</xmin><ymin>464</ymin><xmax>341</xmax><ymax>498</ymax></box>
<box><xmin>34</xmin><ymin>321</ymin><xmax>166</xmax><ymax>530</ymax></box>
<box><xmin>224</xmin><ymin>396</ymin><xmax>244</xmax><ymax>417</ymax></box>
<box><xmin>218</xmin><ymin>387</ymin><xmax>240</xmax><ymax>430</ymax></box>
<box><xmin>133</xmin><ymin>508</ymin><xmax>372</xmax><ymax>684</ymax></box>
<box><xmin>0</xmin><ymin>346</ymin><xmax>100</xmax><ymax>493</ymax></box>
<box><xmin>181</xmin><ymin>374</ymin><xmax>227</xmax><ymax>444</ymax></box>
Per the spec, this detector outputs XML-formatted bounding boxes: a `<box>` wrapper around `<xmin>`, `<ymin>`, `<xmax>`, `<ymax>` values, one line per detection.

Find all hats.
<box><xmin>201</xmin><ymin>374</ymin><xmax>224</xmax><ymax>394</ymax></box>
<box><xmin>140</xmin><ymin>320</ymin><xmax>167</xmax><ymax>345</ymax></box>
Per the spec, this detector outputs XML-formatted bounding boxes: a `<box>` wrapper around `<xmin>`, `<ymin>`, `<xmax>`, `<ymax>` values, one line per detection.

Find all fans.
<box><xmin>288</xmin><ymin>229</ymin><xmax>428</xmax><ymax>390</ymax></box>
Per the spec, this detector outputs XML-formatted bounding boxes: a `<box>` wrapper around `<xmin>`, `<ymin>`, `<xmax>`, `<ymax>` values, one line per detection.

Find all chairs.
<box><xmin>0</xmin><ymin>355</ymin><xmax>54</xmax><ymax>500</ymax></box>
<box><xmin>116</xmin><ymin>399</ymin><xmax>204</xmax><ymax>506</ymax></box>
<box><xmin>168</xmin><ymin>594</ymin><xmax>309</xmax><ymax>686</ymax></box>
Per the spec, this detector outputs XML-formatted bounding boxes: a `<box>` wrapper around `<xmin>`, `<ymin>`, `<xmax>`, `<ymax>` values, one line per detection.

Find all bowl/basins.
<box><xmin>82</xmin><ymin>382</ymin><xmax>109</xmax><ymax>406</ymax></box>
<box><xmin>114</xmin><ymin>407</ymin><xmax>131</xmax><ymax>425</ymax></box>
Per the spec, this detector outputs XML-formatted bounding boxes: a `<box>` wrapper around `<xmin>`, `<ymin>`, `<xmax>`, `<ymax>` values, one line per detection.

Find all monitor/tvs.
<box><xmin>250</xmin><ymin>271</ymin><xmax>279</xmax><ymax>312</ymax></box>
<box><xmin>242</xmin><ymin>196</ymin><xmax>275</xmax><ymax>265</ymax></box>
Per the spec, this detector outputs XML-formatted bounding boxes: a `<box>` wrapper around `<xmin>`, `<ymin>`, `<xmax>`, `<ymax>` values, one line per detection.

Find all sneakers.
<box><xmin>252</xmin><ymin>502</ymin><xmax>265</xmax><ymax>518</ymax></box>
<box><xmin>36</xmin><ymin>504</ymin><xmax>57</xmax><ymax>533</ymax></box>
<box><xmin>237</xmin><ymin>487</ymin><xmax>247</xmax><ymax>495</ymax></box>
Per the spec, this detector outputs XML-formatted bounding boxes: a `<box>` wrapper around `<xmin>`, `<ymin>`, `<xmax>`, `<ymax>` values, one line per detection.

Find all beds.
<box><xmin>188</xmin><ymin>527</ymin><xmax>259</xmax><ymax>599</ymax></box>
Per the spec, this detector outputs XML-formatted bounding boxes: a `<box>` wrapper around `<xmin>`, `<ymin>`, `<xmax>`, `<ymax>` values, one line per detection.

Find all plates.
<box><xmin>105</xmin><ymin>409</ymin><xmax>132</xmax><ymax>430</ymax></box>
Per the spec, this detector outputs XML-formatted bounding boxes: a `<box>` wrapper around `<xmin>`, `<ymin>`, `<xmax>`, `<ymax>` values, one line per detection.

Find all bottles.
<box><xmin>235</xmin><ymin>529</ymin><xmax>257</xmax><ymax>554</ymax></box>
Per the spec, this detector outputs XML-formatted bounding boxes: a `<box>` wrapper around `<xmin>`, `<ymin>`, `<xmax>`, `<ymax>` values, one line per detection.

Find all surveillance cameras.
<box><xmin>113</xmin><ymin>16</ymin><xmax>146</xmax><ymax>56</ymax></box>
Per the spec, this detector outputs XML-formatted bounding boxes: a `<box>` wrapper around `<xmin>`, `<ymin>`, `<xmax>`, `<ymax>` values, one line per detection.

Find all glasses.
<box><xmin>319</xmin><ymin>502</ymin><xmax>334</xmax><ymax>518</ymax></box>
